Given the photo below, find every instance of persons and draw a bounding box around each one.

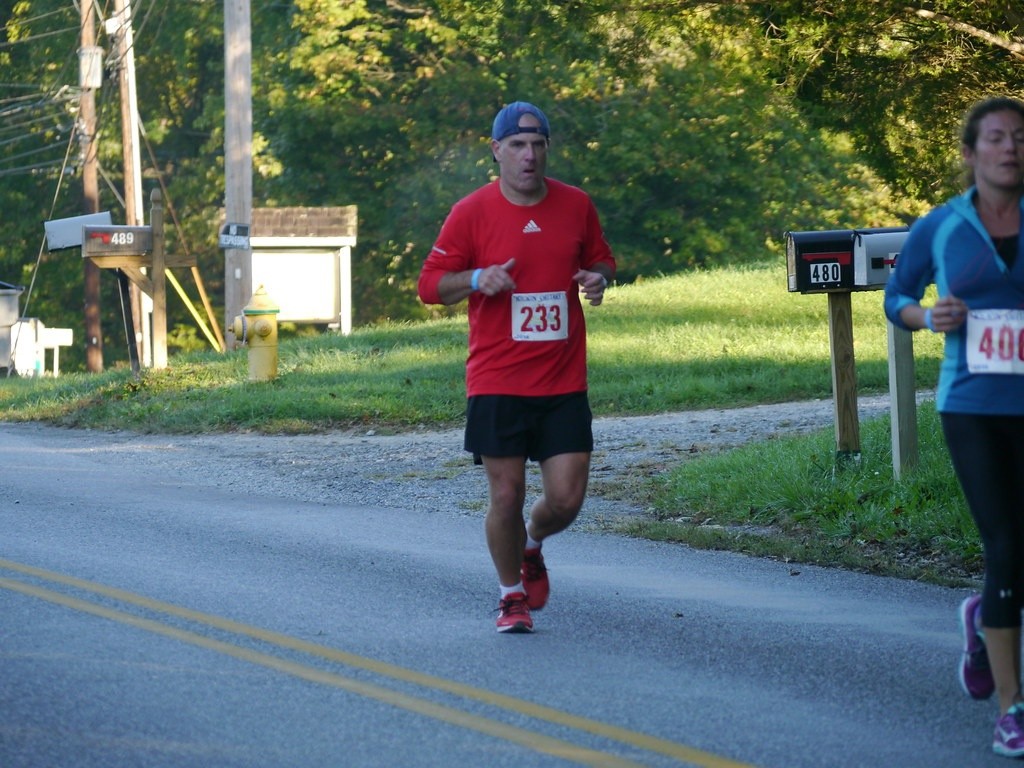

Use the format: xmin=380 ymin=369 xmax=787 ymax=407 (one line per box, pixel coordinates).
xmin=884 ymin=98 xmax=1024 ymax=756
xmin=417 ymin=102 xmax=618 ymax=634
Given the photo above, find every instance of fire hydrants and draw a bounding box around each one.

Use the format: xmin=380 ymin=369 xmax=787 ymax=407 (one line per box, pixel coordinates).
xmin=226 ymin=283 xmax=280 ymax=385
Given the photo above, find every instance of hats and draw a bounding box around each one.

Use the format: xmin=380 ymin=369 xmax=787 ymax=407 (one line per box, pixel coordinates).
xmin=491 ymin=101 xmax=549 ymax=163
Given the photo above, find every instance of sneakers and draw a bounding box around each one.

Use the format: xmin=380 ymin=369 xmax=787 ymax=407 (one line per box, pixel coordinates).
xmin=992 ymin=691 xmax=1024 ymax=757
xmin=959 ymin=595 xmax=996 ymax=700
xmin=520 ymin=547 xmax=550 ymax=611
xmin=489 ymin=592 xmax=533 ymax=633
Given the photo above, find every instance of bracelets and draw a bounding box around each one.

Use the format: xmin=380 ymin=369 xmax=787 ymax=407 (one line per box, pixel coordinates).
xmin=925 ymin=309 xmax=932 ymax=329
xmin=471 ymin=268 xmax=482 ymax=290
